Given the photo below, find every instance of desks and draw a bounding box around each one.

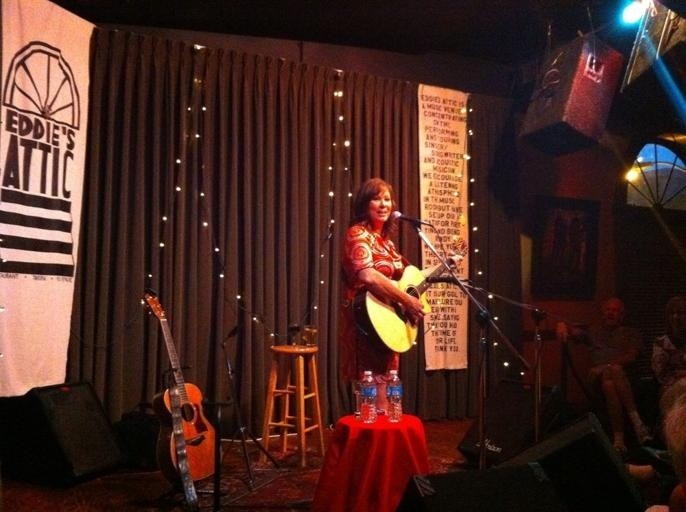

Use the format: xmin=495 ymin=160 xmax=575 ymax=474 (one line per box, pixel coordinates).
xmin=335 ymin=412 xmax=428 ymax=511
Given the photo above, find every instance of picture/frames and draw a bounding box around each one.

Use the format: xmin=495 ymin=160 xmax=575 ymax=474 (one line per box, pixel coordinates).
xmin=531 ymin=194 xmax=600 ymax=301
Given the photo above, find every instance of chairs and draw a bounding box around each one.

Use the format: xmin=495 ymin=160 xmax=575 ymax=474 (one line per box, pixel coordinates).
xmin=564 ymin=337 xmax=606 ymax=410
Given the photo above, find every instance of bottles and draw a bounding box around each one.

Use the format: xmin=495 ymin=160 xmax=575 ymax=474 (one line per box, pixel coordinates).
xmin=385 ymin=369 xmax=404 ymax=424
xmin=359 ymin=371 xmax=378 ymax=425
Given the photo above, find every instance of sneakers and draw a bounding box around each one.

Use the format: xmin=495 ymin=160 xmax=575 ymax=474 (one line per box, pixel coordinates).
xmin=611 ymin=427 xmax=654 ymax=466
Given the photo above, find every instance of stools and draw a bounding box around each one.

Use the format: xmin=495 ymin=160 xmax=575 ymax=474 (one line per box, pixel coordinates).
xmin=255 ymin=343 xmax=327 ymax=468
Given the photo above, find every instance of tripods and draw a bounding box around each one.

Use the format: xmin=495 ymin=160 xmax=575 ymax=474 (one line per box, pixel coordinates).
xmin=219 ymin=325 xmax=281 ymax=488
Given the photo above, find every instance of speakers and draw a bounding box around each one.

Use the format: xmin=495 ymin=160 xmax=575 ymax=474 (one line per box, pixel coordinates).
xmin=488 ymin=412 xmax=646 ymax=512
xmin=458 ymin=384 xmax=580 ymax=470
xmin=0 ymin=382 xmax=136 ymax=490
xmin=519 ymin=31 xmax=626 ymax=158
xmin=396 ymin=460 xmax=565 ymax=512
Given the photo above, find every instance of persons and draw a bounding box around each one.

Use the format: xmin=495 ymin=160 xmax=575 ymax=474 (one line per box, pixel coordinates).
xmin=568 ymin=295 xmax=686 ymax=512
xmin=338 ymin=178 xmax=426 ymax=412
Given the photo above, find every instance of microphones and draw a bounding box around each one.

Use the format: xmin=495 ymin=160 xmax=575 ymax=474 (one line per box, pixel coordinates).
xmin=426 ymin=276 xmax=456 ymax=282
xmin=391 ymin=210 xmax=420 ymax=223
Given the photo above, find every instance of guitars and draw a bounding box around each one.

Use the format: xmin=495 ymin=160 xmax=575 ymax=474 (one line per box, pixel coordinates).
xmin=140 ymin=287 xmax=223 ymax=487
xmin=351 ymin=237 xmax=468 ymax=354
